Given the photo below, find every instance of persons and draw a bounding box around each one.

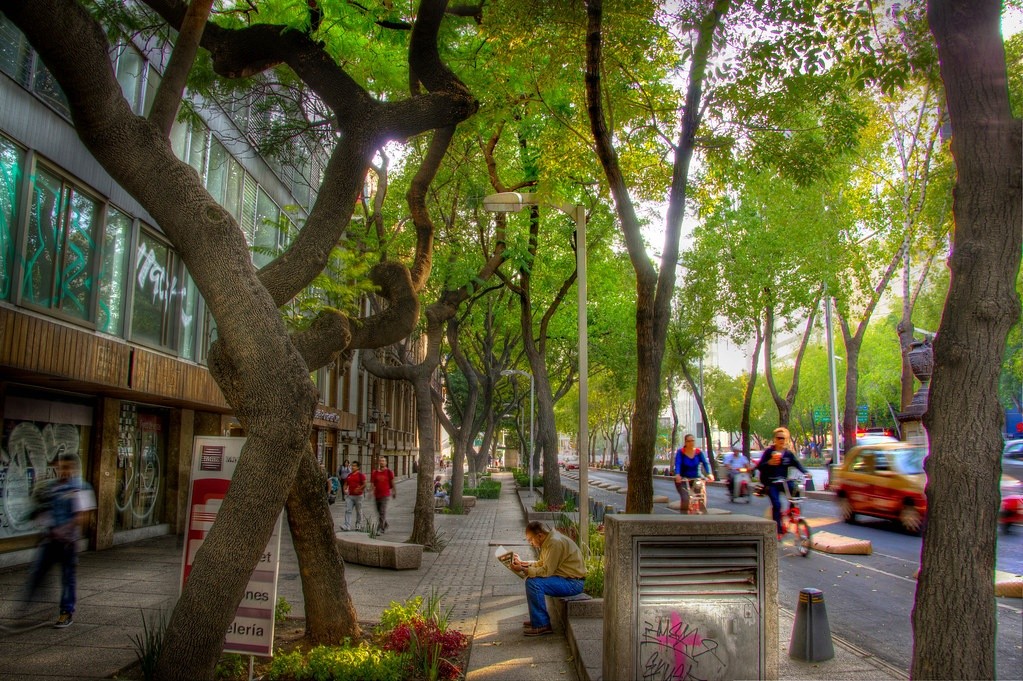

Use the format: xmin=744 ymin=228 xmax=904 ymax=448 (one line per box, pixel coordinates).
xmin=722 ymin=447 xmax=753 ymax=494
xmin=320 ymin=461 xmax=332 ymax=504
xmin=340 ymin=460 xmax=367 ymax=531
xmin=510 ymin=521 xmax=587 ymax=637
xmin=1 ymin=451 xmax=97 ymax=633
xmin=674 ymin=434 xmax=715 ymax=513
xmin=367 ymin=456 xmax=396 ymax=537
xmin=336 ymin=460 xmax=352 ymax=502
xmin=434 ymin=459 xmax=451 ymax=497
xmin=759 ymin=428 xmax=812 ymax=533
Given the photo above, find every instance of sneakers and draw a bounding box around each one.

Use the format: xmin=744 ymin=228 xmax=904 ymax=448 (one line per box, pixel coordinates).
xmin=56 ymin=608 xmax=74 ymax=627
xmin=523 ymin=618 xmax=552 ymax=636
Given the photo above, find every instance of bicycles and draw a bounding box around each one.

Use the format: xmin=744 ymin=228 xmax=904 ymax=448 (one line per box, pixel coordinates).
xmin=764 ymin=474 xmax=815 ymax=558
xmin=679 ymin=477 xmax=711 ymax=517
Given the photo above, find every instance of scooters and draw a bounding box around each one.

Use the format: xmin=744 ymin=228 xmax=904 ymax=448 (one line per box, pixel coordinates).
xmin=724 ymin=463 xmax=754 ymax=503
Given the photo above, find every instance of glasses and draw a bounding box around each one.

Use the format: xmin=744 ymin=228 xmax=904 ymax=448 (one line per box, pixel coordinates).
xmin=776 ymin=436 xmax=786 ymax=440
xmin=686 ymin=440 xmax=694 ymax=443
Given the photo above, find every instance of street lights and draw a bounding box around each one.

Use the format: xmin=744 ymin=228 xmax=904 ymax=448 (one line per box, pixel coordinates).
xmin=502 ymin=414 xmax=520 ymax=474
xmin=484 ymin=190 xmax=590 ymax=562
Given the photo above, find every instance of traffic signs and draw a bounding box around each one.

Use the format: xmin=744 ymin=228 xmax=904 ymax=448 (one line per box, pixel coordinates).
xmin=812 ymin=402 xmax=869 ymax=423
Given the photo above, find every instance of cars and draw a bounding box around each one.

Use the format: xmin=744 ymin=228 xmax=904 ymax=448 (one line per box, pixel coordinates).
xmin=855 ymin=435 xmax=901 ymax=469
xmin=562 ymin=455 xmax=579 ymax=471
xmin=748 ymin=450 xmax=767 ymax=471
xmin=1001 ymin=438 xmax=1023 ymax=486
xmin=558 ymin=456 xmax=566 ymax=467
xmin=829 ymin=441 xmax=1023 ymax=539
xmin=701 ymin=451 xmax=745 ymax=481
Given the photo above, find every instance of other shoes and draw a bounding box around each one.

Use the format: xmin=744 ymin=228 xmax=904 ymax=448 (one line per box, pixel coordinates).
xmin=355 ymin=523 xmax=360 ymax=531
xmin=340 ymin=523 xmax=349 ymax=530
xmin=376 ymin=522 xmax=389 ymax=536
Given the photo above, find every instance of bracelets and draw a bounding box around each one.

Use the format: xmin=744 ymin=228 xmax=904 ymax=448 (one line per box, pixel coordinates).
xmin=521 ymin=567 xmax=525 ymax=571
